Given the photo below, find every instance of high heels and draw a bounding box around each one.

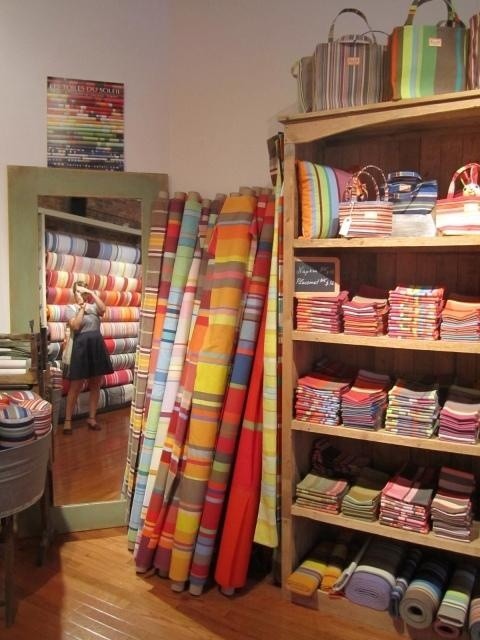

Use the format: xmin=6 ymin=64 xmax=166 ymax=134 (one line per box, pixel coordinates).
xmin=63 ymin=420 xmax=73 ymax=435
xmin=87 ymin=417 xmax=101 ymax=431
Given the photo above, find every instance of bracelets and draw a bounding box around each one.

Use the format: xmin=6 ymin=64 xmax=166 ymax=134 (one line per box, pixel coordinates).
xmin=77 ymin=313 xmax=84 ymax=316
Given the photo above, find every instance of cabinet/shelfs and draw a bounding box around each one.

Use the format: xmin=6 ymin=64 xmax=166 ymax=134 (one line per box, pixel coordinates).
xmin=279 ymin=88 xmax=480 ymax=637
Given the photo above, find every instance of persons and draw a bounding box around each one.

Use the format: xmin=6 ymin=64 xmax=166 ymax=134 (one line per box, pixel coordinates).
xmin=61 ymin=278 xmax=115 ymax=434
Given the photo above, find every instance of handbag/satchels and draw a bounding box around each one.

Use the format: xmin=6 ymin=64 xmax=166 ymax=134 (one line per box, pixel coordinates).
xmin=291 ymin=56 xmax=313 ymax=113
xmin=388 ymin=0 xmax=471 ymax=101
xmin=312 ymin=8 xmax=393 ymax=112
xmin=467 ymin=12 xmax=480 ymax=90
xmin=61 ymin=327 xmax=74 ymax=365
xmin=435 ymin=163 xmax=480 ymax=236
xmin=338 ymin=165 xmax=393 ymax=238
xmin=378 ymin=171 xmax=438 ymax=214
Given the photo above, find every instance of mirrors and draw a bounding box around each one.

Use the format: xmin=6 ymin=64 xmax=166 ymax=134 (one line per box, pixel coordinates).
xmin=7 ymin=164 xmax=169 ymax=536
